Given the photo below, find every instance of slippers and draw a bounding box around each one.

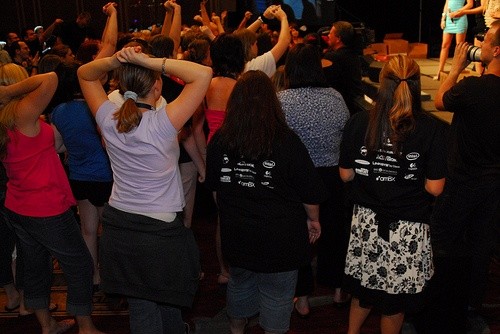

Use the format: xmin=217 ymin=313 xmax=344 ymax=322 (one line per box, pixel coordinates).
xmin=293 ymin=298 xmax=308 ymax=319
xmin=325 ymin=294 xmax=343 ymax=309
xmin=17 ymin=303 xmax=59 ymax=321
xmin=5 ymin=300 xmax=22 ymax=311
xmin=44 ymin=318 xmax=76 ymax=334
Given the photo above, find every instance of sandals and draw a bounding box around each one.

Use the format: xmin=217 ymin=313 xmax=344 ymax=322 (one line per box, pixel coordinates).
xmin=216 ymin=273 xmax=231 ymax=288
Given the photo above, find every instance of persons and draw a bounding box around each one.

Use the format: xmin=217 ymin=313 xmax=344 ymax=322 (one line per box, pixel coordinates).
xmin=0 ymin=0 xmax=500 ymax=334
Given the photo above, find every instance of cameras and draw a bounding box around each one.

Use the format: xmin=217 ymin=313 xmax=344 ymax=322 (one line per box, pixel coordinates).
xmin=466 ymin=32 xmax=486 ymax=62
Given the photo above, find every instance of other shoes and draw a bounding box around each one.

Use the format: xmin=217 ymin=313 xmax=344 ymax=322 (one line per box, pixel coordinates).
xmin=91 ymin=283 xmax=103 ymax=294
